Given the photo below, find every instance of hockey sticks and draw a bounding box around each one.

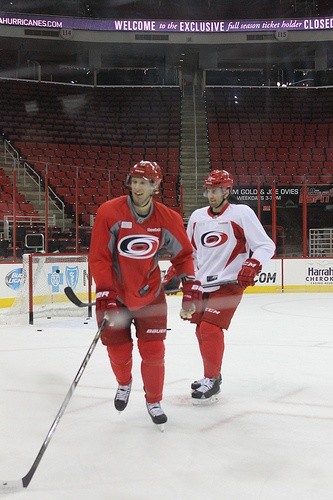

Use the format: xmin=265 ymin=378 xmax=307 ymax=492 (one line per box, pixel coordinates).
xmin=64 ymin=278 xmax=240 ymax=308
xmin=0 ymin=314 xmax=110 ymax=490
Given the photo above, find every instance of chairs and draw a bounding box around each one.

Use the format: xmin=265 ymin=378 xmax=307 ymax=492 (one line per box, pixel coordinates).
xmin=204 ymin=88 xmax=333 ymax=186
xmin=0 ymin=79 xmax=183 ymax=252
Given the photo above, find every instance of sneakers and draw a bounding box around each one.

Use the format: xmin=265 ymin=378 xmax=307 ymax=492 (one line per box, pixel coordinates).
xmin=114 ymin=376 xmax=134 ymax=413
xmin=192 ymin=378 xmax=222 ymax=404
xmin=146 ymin=400 xmax=168 ymax=431
xmin=190 ymin=373 xmax=223 ymax=390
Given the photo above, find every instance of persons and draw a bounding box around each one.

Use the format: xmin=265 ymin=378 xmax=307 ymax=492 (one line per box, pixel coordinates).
xmin=88 ymin=160 xmax=203 ymax=424
xmin=161 ymin=169 xmax=276 ymax=401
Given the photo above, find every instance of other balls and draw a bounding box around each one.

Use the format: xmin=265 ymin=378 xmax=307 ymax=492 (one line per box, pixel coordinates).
xmin=47 ymin=316 xmax=51 ymax=319
xmin=37 ymin=329 xmax=42 ymax=331
xmin=84 ymin=321 xmax=88 ymax=324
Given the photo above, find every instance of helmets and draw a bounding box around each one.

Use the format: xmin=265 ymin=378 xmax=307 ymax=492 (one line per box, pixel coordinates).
xmin=203 ymin=170 xmax=233 ymax=193
xmin=127 ymin=160 xmax=162 ymax=185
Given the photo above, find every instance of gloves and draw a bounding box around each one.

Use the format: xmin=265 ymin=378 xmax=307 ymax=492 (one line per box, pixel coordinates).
xmin=162 ymin=265 xmax=180 ymax=295
xmin=180 ymin=280 xmax=202 ymax=319
xmin=237 ymin=257 xmax=262 ymax=290
xmin=96 ymin=291 xmax=117 ymax=328
